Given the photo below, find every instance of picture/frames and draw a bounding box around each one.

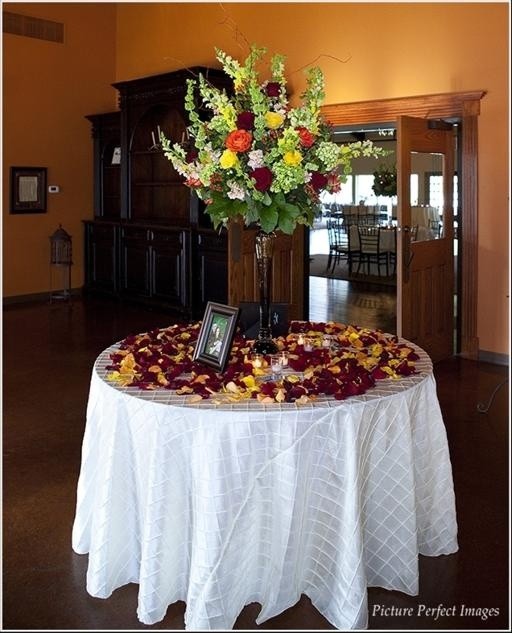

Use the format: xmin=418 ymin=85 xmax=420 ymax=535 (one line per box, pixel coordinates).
xmin=9 ymin=166 xmax=48 ymax=216
xmin=190 ymin=302 xmax=240 ymax=374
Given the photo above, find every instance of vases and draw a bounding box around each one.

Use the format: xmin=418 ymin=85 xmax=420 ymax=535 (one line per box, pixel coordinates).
xmin=253 ymin=230 xmax=278 ymax=352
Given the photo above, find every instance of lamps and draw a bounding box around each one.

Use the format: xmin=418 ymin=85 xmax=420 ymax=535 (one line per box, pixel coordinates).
xmin=47 ymin=226 xmax=81 ymax=304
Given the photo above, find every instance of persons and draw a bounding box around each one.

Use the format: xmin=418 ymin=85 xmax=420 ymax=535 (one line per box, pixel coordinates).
xmin=207 ymin=322 xmax=223 ymax=345
xmin=209 ymin=326 xmax=223 ymax=354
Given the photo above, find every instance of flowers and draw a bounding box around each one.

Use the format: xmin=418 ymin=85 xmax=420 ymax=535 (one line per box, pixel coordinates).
xmin=151 ymin=37 xmax=396 ymax=239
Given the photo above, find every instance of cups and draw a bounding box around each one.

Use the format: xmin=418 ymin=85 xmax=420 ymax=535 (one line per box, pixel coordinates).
xmin=251 ymin=350 xmax=290 ymax=374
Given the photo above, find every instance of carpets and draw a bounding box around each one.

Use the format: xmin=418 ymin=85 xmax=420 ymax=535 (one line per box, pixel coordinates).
xmin=308 ymin=253 xmax=395 ymax=286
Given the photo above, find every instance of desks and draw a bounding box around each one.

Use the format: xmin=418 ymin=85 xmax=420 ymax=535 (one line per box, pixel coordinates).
xmin=89 ymin=321 xmax=442 ymax=628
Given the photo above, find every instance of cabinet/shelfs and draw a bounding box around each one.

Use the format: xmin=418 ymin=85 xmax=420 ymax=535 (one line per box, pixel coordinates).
xmin=79 ymin=66 xmax=234 ymax=316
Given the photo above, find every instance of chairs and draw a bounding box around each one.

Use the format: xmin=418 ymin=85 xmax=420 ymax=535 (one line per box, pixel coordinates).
xmin=315 ymin=199 xmax=442 ymax=278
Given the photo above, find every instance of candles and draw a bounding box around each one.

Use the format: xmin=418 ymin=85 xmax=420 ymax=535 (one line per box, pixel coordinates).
xmin=294 ymin=330 xmax=332 ymax=351
xmin=252 ymin=350 xmax=292 ymax=374
